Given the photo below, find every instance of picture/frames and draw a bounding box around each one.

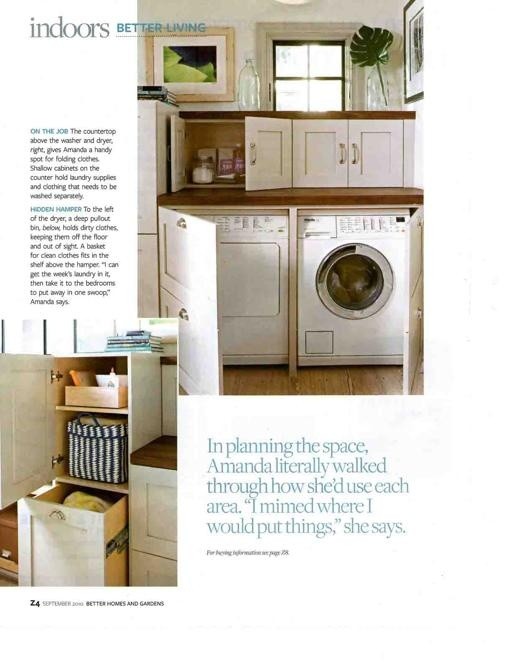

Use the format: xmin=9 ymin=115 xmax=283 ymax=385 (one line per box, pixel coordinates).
xmin=400 ymin=0 xmax=425 ymax=106
xmin=143 ymin=21 xmax=238 ymax=104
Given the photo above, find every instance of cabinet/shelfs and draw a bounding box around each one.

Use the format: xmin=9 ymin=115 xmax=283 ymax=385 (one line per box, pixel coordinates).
xmin=126 ymin=467 xmax=177 ymax=587
xmin=0 ymin=351 xmax=164 ymax=511
xmin=164 ymin=112 xmax=292 ymax=193
xmin=157 ymin=203 xmax=425 ymax=396
xmin=15 ymin=482 xmax=129 ymax=587
xmin=290 ymin=118 xmax=405 ymax=189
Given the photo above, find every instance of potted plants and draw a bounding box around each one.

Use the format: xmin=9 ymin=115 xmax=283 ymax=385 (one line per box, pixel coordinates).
xmin=348 ymin=23 xmax=395 ymax=110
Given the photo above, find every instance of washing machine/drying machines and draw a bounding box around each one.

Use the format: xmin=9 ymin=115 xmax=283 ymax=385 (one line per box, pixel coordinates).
xmin=159 ymin=208 xmax=291 ymax=394
xmin=298 ymin=212 xmax=411 ymax=369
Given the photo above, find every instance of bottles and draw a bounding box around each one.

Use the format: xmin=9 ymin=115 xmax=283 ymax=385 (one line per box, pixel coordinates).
xmin=192 ymin=154 xmax=215 ymax=184
xmin=233 ymin=144 xmax=246 ymax=184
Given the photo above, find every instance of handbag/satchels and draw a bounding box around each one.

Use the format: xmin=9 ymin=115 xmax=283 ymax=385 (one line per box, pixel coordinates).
xmin=66 ymin=411 xmax=128 ymax=485
